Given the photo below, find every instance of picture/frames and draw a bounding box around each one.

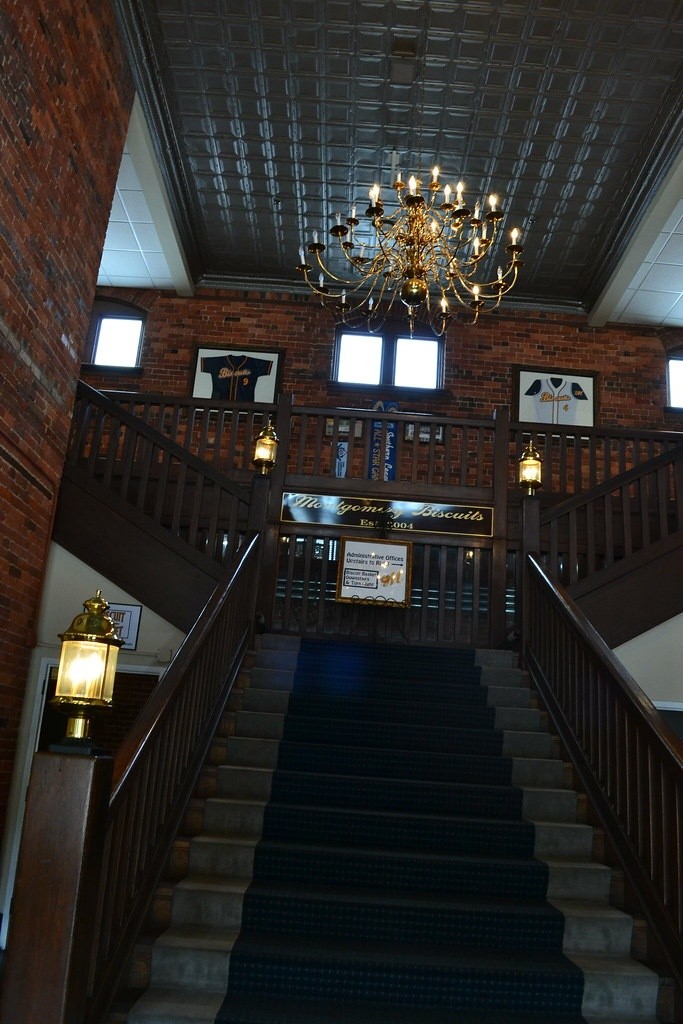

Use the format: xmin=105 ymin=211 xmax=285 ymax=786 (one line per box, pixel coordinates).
xmin=83 ymin=602 xmax=142 ymax=651
xmin=181 ymin=342 xmax=288 ymax=426
xmin=511 ymin=362 xmax=602 ymax=447
xmin=334 ymin=536 xmax=414 ymax=609
xmin=403 ymin=411 xmax=446 ymax=445
xmin=322 ymin=407 xmax=366 ymax=441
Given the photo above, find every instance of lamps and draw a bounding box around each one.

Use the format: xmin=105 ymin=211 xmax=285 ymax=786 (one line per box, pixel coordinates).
xmin=252 ymin=419 xmax=280 ymax=476
xmin=296 ymin=2 xmax=527 ymax=340
xmin=49 ymin=588 xmax=127 ymax=756
xmin=517 ymin=440 xmax=547 ymax=497
xmin=464 ymin=549 xmax=474 ymax=569
